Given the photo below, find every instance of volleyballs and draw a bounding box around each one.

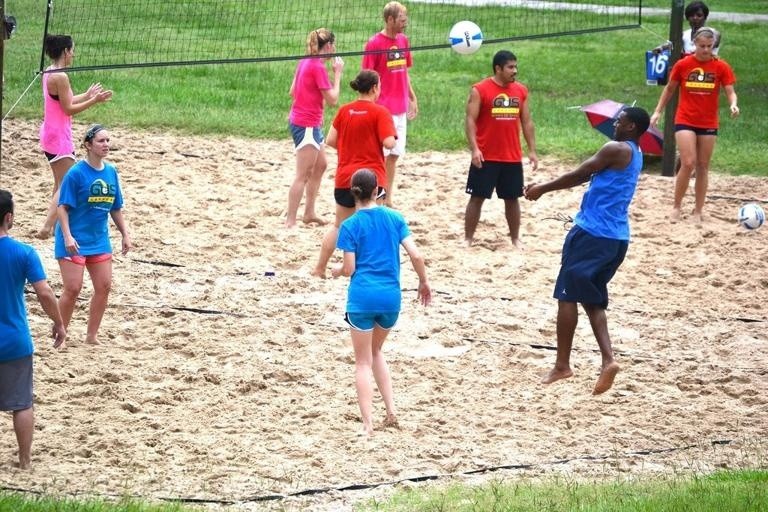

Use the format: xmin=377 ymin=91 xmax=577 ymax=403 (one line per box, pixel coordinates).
xmin=449 ymin=20 xmax=484 ymax=56
xmin=738 ymin=203 xmax=765 ymax=230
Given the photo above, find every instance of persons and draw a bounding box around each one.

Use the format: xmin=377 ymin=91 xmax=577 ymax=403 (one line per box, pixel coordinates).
xmin=331 ymin=167 xmax=432 ymax=438
xmin=35 ymin=33 xmax=113 ymax=237
xmin=463 ymin=50 xmax=538 ymax=253
xmin=521 ymin=104 xmax=649 ymax=397
xmin=363 ymin=1 xmax=418 ymax=208
xmin=652 ymin=2 xmax=721 ymax=175
xmin=284 ymin=27 xmax=345 ymax=231
xmin=0 ymin=189 xmax=67 ymax=470
xmin=49 ymin=122 xmax=131 ymax=346
xmin=308 ymin=69 xmax=398 ymax=289
xmin=649 ymin=26 xmax=740 ymax=225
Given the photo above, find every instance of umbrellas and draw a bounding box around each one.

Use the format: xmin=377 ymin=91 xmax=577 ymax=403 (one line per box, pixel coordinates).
xmin=578 ymin=98 xmax=665 ymax=157
